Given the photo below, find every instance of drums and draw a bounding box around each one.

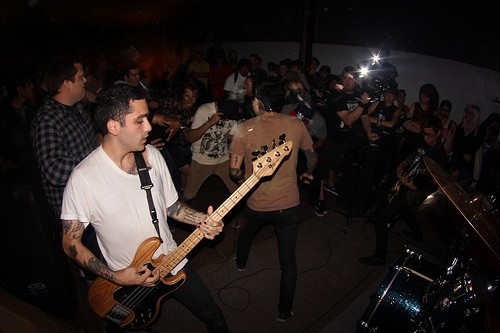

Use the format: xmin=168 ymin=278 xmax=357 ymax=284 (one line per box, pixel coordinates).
xmin=425 ymin=260 xmax=496 ymax=333
xmin=358 ymin=248 xmax=450 ymax=333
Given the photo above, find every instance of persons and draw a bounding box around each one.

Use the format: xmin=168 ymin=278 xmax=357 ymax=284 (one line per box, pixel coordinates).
xmin=31 ymin=61 xmax=107 ymax=333
xmin=311 ymin=47 xmax=500 ymax=217
xmin=60 ymin=83 xmax=224 ymax=333
xmin=227 ymin=77 xmax=317 ymax=323
xmin=112 ymin=55 xmax=331 ymax=211
xmin=0 ymin=53 xmax=105 ymax=271
xmin=182 ymin=79 xmax=238 ymax=202
xmin=359 ymin=115 xmax=446 ymax=265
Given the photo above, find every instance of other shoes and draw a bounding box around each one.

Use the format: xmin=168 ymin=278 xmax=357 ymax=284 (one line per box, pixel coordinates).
xmin=276 ymin=311 xmax=294 ymax=322
xmin=234 ymin=257 xmax=247 ymax=272
xmin=360 ymin=255 xmax=384 ymax=264
xmin=312 ymin=205 xmax=327 ymax=216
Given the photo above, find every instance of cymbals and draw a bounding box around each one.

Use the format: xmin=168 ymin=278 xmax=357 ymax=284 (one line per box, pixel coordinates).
xmin=423 ymin=155 xmax=500 ymax=260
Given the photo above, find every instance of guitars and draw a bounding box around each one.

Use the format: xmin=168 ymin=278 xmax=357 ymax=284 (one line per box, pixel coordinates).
xmin=89 ymin=133 xmax=294 ymax=329
xmin=364 ymin=149 xmax=427 ymax=219
